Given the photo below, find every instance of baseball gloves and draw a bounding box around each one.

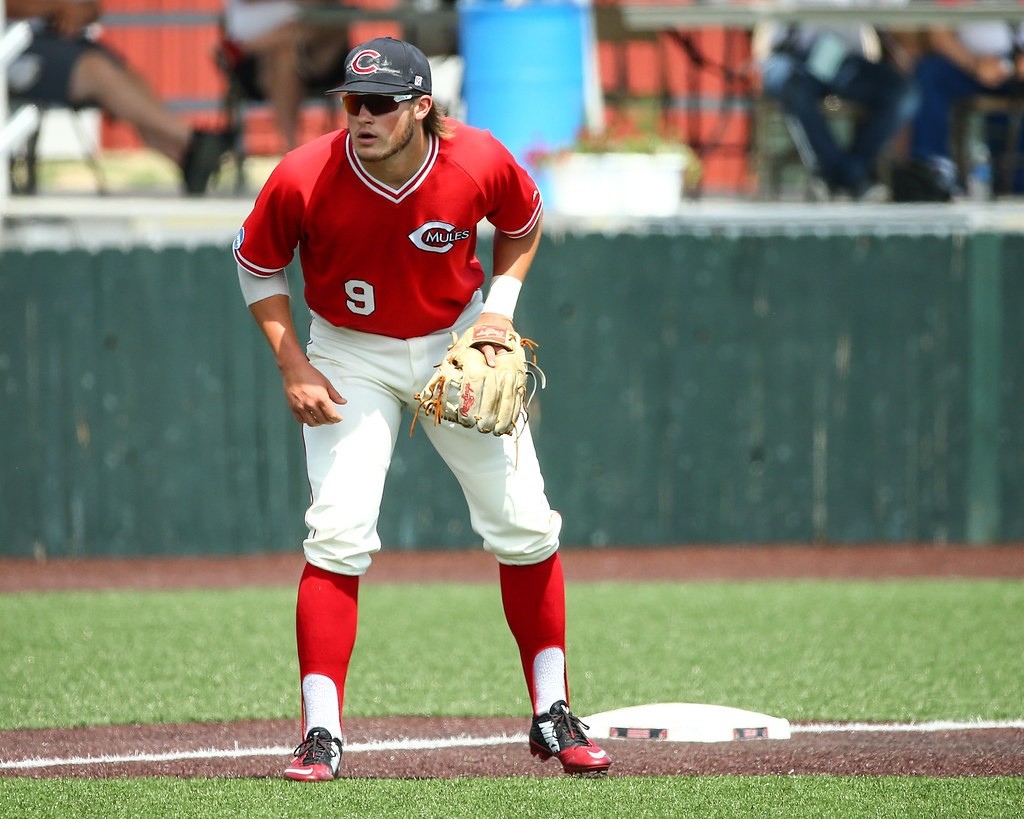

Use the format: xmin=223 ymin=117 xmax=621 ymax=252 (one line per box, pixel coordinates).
xmin=420 ymin=324 xmax=529 ymax=437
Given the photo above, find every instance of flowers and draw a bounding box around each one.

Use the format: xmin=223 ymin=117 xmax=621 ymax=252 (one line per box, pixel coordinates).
xmin=529 ymin=102 xmax=696 ymax=181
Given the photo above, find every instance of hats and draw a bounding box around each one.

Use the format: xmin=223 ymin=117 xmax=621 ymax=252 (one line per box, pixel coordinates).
xmin=324 ymin=37 xmax=432 ymax=97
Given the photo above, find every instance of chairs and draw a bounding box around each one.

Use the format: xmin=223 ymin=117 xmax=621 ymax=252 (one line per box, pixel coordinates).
xmin=9 ymin=90 xmax=105 ymax=199
xmin=221 ymin=85 xmax=343 ymax=201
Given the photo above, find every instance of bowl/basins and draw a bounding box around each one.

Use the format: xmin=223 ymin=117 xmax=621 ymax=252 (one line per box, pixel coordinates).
xmin=544 ymin=151 xmax=683 ymax=221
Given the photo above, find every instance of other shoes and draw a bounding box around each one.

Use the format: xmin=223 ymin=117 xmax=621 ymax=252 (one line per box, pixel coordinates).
xmin=180 ymin=121 xmax=239 ymax=194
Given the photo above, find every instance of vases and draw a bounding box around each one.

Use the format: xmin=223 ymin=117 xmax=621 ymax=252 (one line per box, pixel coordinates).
xmin=556 ymin=152 xmax=693 ymax=219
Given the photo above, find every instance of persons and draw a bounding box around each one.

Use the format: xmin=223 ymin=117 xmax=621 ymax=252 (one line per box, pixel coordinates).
xmin=750 ymin=0 xmax=1023 ymax=204
xmin=232 ymin=37 xmax=611 ymax=782
xmin=5 ymin=2 xmax=459 ymax=195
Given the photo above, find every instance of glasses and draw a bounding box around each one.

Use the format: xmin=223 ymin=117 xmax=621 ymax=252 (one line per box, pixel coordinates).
xmin=341 ymin=93 xmax=426 ymax=116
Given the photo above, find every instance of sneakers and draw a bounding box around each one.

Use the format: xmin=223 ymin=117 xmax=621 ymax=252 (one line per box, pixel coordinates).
xmin=283 ymin=727 xmax=343 ymax=782
xmin=529 ymin=699 xmax=612 ymax=776
